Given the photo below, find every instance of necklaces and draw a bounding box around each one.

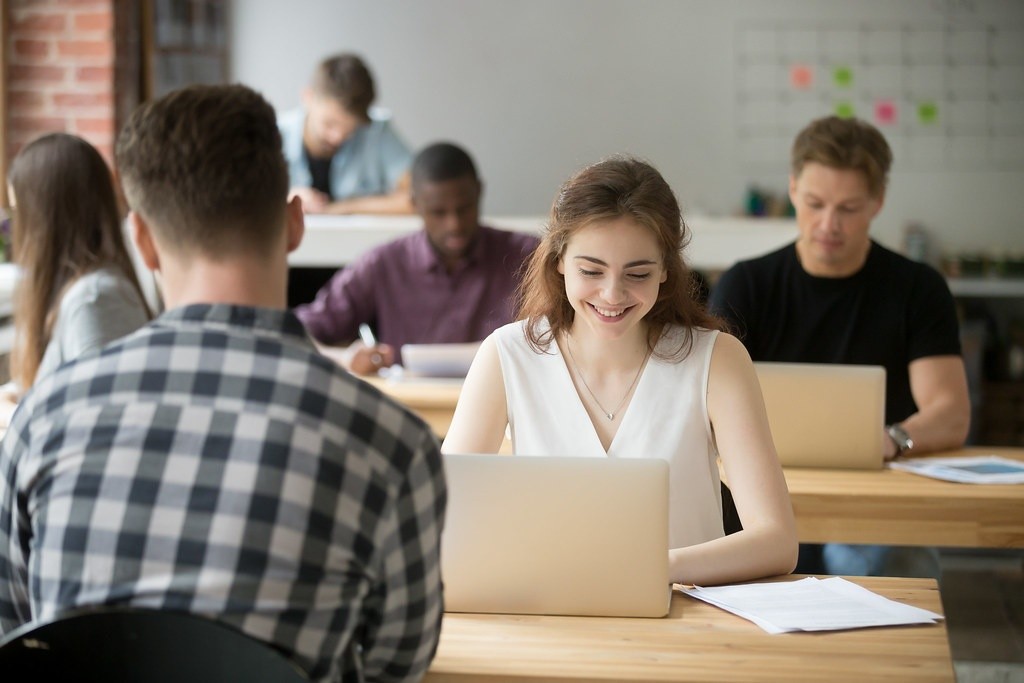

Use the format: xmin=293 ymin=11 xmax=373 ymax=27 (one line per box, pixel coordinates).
xmin=567 ymin=329 xmax=649 ymax=420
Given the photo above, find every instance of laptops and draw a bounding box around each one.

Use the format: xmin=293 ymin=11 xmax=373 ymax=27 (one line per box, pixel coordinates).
xmin=439 ymin=454 xmax=673 ymax=618
xmin=752 ymin=361 xmax=888 ymax=469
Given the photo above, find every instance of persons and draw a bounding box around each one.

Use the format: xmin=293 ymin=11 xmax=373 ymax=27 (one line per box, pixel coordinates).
xmin=276 ymin=55 xmax=416 ymax=215
xmin=712 ymin=115 xmax=970 ymax=575
xmin=0 ymin=83 xmax=447 ymax=683
xmin=8 ymin=133 xmax=151 ymax=403
xmin=439 ymin=157 xmax=799 ymax=583
xmin=297 ymin=141 xmax=541 ymax=378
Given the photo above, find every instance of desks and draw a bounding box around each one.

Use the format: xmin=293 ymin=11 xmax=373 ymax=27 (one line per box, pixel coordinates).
xmin=289 ymin=216 xmax=1024 ymax=683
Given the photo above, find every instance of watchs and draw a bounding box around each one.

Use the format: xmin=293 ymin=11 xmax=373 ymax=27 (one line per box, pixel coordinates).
xmin=884 ymin=425 xmax=910 ymax=462
